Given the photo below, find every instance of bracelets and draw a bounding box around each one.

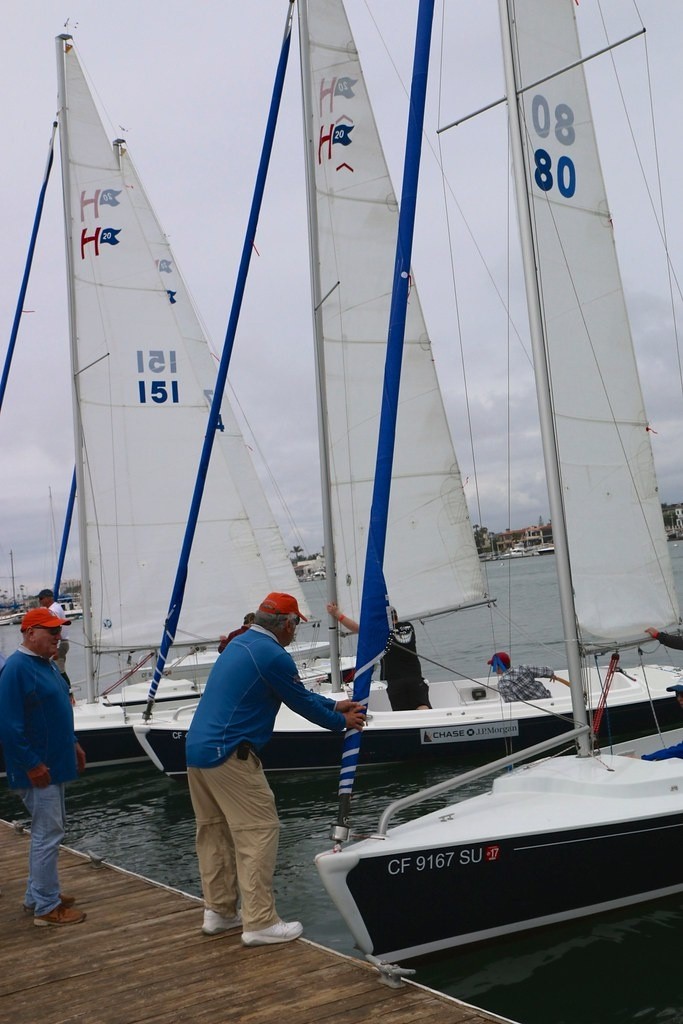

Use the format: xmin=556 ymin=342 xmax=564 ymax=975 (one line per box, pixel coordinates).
xmin=338 ymin=614 xmax=345 ymax=621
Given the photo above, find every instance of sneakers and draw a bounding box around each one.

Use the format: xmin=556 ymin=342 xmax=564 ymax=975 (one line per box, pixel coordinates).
xmin=241 ymin=918 xmax=303 ymax=946
xmin=23 ymin=894 xmax=75 ymax=913
xmin=202 ymin=909 xmax=242 ymax=934
xmin=34 ymin=907 xmax=86 ymax=926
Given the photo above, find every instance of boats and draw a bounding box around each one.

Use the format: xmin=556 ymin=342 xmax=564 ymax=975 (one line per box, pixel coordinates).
xmin=537 ymin=546 xmax=554 ymax=556
xmin=500 ymin=553 xmax=510 ymax=559
xmin=509 ymin=546 xmax=532 ymax=557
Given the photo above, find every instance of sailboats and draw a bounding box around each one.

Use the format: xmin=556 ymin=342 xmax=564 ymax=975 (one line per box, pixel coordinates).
xmin=1 ymin=0 xmax=683 ymax=972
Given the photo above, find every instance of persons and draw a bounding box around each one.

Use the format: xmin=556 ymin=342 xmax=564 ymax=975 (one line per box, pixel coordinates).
xmin=644 ymin=626 xmax=683 ymax=650
xmin=187 ymin=592 xmax=368 ymax=945
xmin=487 ymin=652 xmax=555 ymax=702
xmin=628 ymin=676 xmax=683 ymax=762
xmin=327 ymin=602 xmax=432 ymax=711
xmin=35 ymin=590 xmax=75 ymax=705
xmin=0 ymin=608 xmax=86 ymax=928
xmin=218 ymin=613 xmax=255 ymax=653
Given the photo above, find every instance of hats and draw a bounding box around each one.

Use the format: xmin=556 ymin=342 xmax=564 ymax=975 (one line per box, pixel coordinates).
xmin=487 ymin=652 xmax=510 ymax=665
xmin=259 ymin=593 xmax=308 ymax=623
xmin=20 ymin=608 xmax=72 ymax=631
xmin=33 ymin=589 xmax=54 ymax=598
xmin=666 ymin=676 xmax=683 ymax=692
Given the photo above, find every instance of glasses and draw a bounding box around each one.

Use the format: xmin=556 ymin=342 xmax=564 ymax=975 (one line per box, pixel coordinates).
xmin=32 ymin=626 xmax=62 ymax=635
xmin=39 ymin=597 xmax=45 ymax=600
